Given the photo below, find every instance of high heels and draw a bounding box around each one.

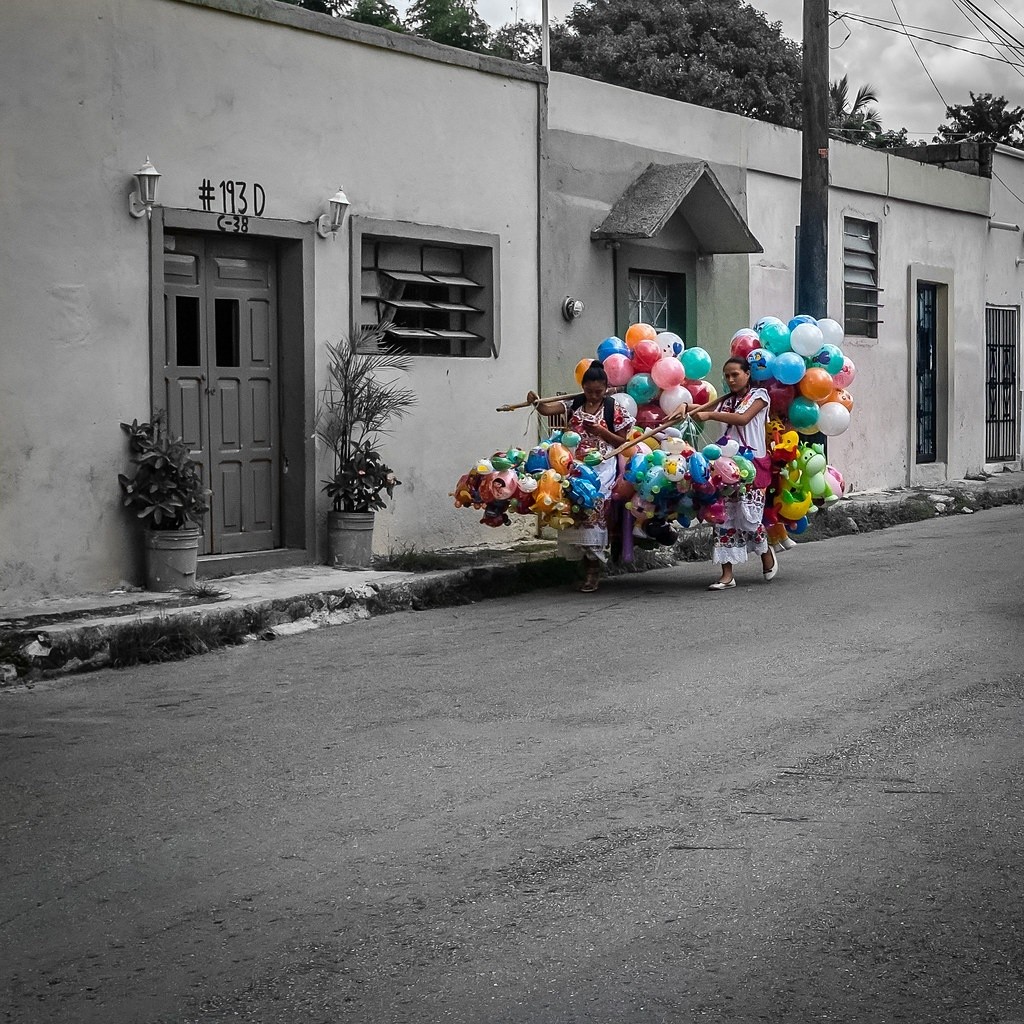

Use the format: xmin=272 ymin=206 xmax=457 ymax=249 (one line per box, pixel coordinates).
xmin=574 ymin=567 xmax=601 ymax=593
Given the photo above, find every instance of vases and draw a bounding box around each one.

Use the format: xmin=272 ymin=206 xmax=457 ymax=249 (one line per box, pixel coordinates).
xmin=146 ymin=529 xmax=200 ymax=592
xmin=327 ymin=510 xmax=375 ymax=569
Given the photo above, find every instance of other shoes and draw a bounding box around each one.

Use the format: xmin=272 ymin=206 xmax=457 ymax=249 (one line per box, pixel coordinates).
xmin=763 ymin=546 xmax=779 ymax=581
xmin=708 ymin=577 xmax=737 ymax=590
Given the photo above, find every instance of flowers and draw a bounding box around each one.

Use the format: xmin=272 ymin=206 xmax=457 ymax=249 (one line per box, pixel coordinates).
xmin=117 ymin=407 xmax=214 ymax=530
xmin=308 ymin=322 xmax=419 ymax=512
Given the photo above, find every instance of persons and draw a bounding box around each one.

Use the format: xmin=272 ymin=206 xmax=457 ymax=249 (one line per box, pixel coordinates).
xmin=663 ymin=356 xmax=778 ymax=590
xmin=526 ymin=360 xmax=636 ymax=592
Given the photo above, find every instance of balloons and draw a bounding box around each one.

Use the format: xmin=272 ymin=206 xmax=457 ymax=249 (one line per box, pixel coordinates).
xmin=729 ymin=313 xmax=857 ymax=436
xmin=575 ymin=322 xmax=717 ymax=435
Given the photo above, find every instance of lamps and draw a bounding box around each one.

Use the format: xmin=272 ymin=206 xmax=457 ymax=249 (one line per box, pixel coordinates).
xmin=316 ymin=183 xmax=350 ymax=238
xmin=129 ymin=154 xmax=162 ymax=219
xmin=563 ymin=295 xmax=585 ymax=322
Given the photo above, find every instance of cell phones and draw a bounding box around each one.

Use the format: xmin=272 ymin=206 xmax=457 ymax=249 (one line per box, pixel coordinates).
xmin=583 ymin=420 xmax=594 ymax=430
